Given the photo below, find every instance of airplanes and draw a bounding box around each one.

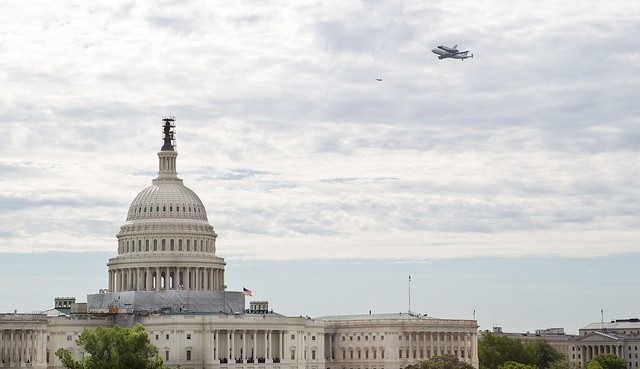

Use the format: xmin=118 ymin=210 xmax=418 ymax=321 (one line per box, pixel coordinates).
xmin=432 ymin=44 xmax=473 ymax=61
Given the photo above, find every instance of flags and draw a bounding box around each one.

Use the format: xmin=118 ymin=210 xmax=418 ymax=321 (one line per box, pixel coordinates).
xmin=243 ymin=287 xmax=252 ymax=296
